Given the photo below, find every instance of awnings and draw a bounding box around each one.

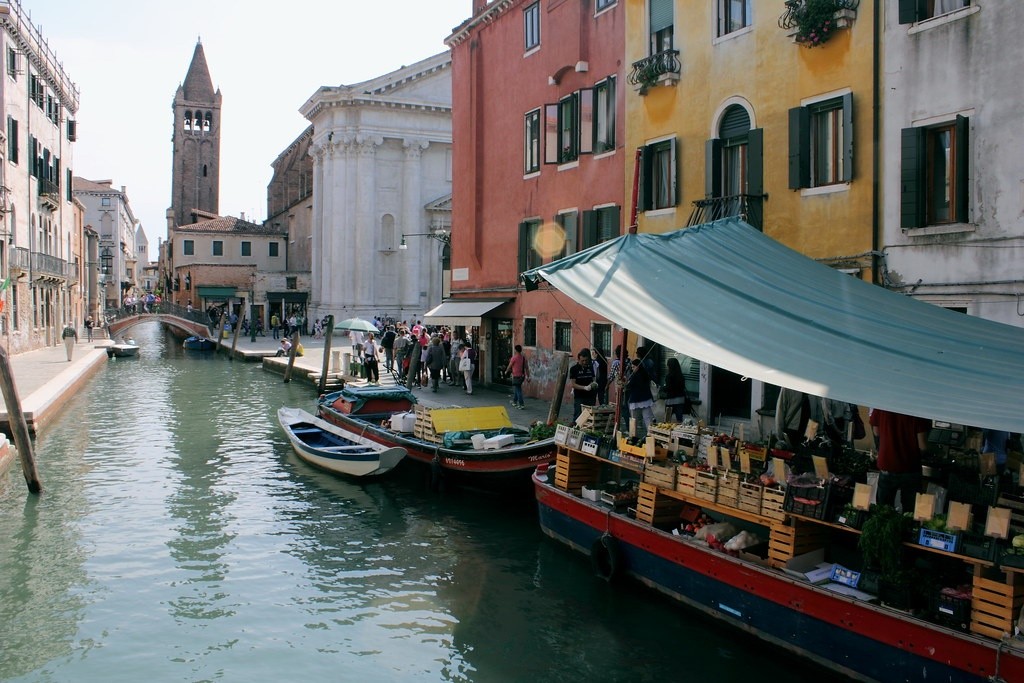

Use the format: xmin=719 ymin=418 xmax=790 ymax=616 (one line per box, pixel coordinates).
xmin=422 ymin=302 xmax=505 ymax=327
xmin=196 ymin=286 xmax=235 ymax=299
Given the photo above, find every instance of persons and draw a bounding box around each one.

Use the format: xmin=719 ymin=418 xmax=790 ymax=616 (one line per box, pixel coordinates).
xmin=84 ymin=292 xmax=329 ymax=358
xmin=504 ymin=345 xmax=531 ymax=410
xmin=568 ymin=345 xmax=686 ymax=442
xmin=348 ymin=315 xmax=477 ymax=396
xmin=775 ymin=387 xmax=1024 ymax=520
xmin=62 ymin=322 xmax=78 ymax=361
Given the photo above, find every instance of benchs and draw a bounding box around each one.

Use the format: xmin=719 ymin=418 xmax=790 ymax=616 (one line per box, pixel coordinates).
xmin=293 ymin=429 xmax=323 ymax=434
xmin=317 ymin=445 xmax=371 ymax=451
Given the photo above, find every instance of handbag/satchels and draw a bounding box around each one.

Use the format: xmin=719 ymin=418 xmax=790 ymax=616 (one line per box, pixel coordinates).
xmin=850 ymin=413 xmax=866 ymax=439
xmin=365 ymin=353 xmax=371 ymax=358
xmin=682 ymin=401 xmax=691 ymax=414
xmin=512 ymin=376 xmax=524 ymax=386
xmin=426 ymin=346 xmax=432 ymax=366
xmin=458 ymin=351 xmax=470 ymax=370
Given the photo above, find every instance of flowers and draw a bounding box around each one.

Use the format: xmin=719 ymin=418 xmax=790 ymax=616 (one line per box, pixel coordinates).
xmin=792 ymin=0 xmax=849 ymax=50
xmin=636 ymin=62 xmax=665 ymax=94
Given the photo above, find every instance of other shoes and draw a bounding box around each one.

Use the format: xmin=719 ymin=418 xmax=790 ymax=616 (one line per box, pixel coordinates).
xmin=515 ymin=405 xmax=524 ymax=409
xmin=440 ymin=380 xmax=446 ymax=383
xmin=462 ymin=386 xmax=474 ymax=395
xmin=417 ymin=384 xmax=421 ymax=389
xmin=431 ymin=385 xmax=439 ymax=392
xmin=510 ymin=400 xmax=517 ymax=407
xmin=446 ymin=381 xmax=460 ymax=386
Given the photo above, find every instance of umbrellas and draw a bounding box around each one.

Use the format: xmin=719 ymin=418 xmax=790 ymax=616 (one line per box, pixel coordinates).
xmin=334 ymin=316 xmax=381 ymax=363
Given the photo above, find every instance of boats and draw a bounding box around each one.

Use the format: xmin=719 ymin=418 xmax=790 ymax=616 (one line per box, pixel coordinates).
xmin=277 ymin=403 xmax=408 ymax=478
xmin=106 ymin=344 xmax=139 ymax=358
xmin=318 ymin=386 xmax=557 ymax=477
xmin=531 ymin=406 xmax=1024 ymax=682
xmin=185 ymin=336 xmax=214 ymax=350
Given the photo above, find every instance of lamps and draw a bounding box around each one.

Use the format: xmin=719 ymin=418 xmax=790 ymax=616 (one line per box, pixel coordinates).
xmin=398 ymin=233 xmax=451 ymax=250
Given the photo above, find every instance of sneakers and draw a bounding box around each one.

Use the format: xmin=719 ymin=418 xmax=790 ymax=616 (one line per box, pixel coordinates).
xmin=368 ymin=381 xmax=383 ymax=386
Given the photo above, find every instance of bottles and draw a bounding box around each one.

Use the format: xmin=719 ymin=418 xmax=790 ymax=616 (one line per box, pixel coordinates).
xmin=675 ymin=449 xmax=717 ymax=480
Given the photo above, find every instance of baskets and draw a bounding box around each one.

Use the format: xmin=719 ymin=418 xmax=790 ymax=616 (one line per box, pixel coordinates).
xmin=553 ymin=424 xmax=615 ymax=458
xmin=833 ymin=504 xmax=1024 ymax=575
xmin=946 ymin=472 xmax=1003 ymax=506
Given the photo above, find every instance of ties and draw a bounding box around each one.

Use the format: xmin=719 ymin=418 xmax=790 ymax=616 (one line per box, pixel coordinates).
xmin=797 ymin=393 xmax=810 ymax=437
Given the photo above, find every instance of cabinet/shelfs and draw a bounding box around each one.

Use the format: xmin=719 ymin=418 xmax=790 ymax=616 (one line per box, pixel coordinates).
xmin=580 ymin=401 xmax=617 ymax=433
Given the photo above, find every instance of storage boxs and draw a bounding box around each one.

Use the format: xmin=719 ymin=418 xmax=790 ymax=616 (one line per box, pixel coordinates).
xmin=391 ymin=413 xmax=417 ymax=433
xmin=552 ymin=424 xmax=854 ymax=522
xmin=919 ymin=528 xmax=1024 ymax=568
xmin=484 ymin=434 xmax=515 ymax=450
